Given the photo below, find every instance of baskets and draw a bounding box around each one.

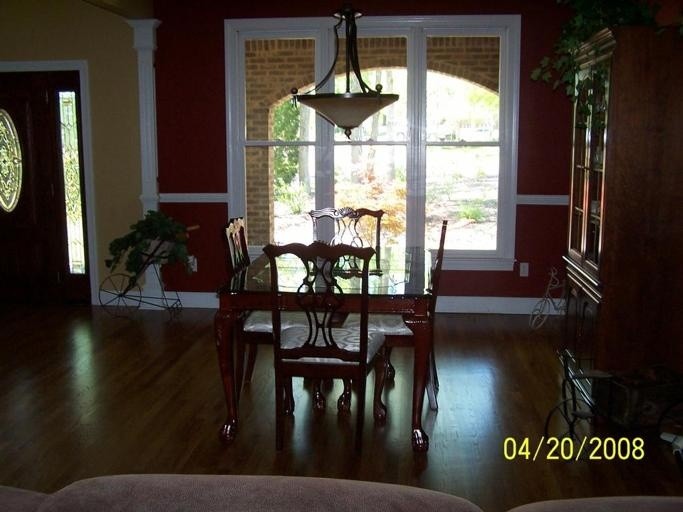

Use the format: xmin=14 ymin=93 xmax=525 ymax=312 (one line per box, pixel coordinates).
xmin=141 ymin=239 xmax=176 ymax=264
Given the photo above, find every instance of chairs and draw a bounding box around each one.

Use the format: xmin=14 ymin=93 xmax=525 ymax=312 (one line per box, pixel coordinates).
xmin=309 ymin=207 xmax=384 ymax=270
xmin=263 ymin=242 xmax=388 ymax=451
xmin=224 ymin=216 xmax=322 ymax=414
xmin=342 ymin=220 xmax=448 ymax=411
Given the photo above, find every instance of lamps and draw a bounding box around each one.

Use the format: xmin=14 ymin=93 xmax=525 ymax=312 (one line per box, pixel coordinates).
xmin=290 ymin=8 xmax=403 ymax=137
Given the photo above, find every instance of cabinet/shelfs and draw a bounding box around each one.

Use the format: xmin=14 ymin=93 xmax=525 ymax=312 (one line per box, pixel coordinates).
xmin=556 ymin=23 xmax=673 ymax=416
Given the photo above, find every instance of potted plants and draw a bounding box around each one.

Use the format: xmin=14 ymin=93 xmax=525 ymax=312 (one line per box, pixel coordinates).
xmin=105 ymin=210 xmax=196 ymax=283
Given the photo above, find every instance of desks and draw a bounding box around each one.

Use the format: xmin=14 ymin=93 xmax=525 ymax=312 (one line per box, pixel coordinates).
xmin=212 ymin=242 xmax=439 ymax=451
